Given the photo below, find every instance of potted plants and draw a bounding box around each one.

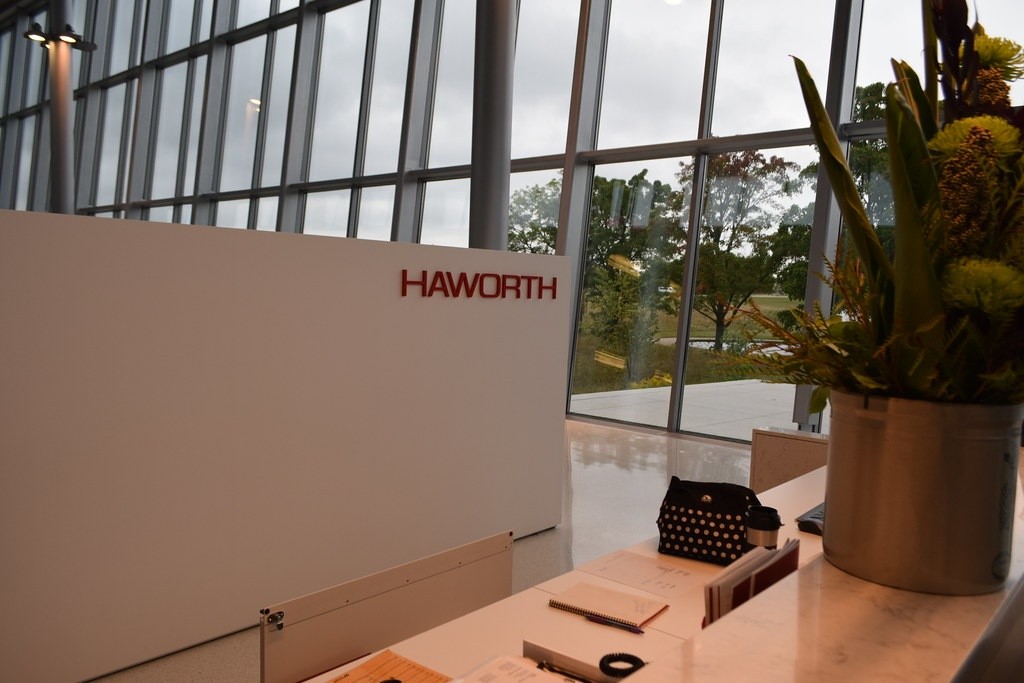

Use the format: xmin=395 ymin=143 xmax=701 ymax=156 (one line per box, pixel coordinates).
xmin=690 ymin=0 xmax=1024 ymax=597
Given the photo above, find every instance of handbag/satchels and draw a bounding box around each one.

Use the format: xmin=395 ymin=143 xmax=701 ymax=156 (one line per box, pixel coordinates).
xmin=656 ymin=476 xmax=763 ymax=567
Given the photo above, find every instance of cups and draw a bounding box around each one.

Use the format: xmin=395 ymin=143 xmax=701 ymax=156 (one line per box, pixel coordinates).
xmin=744 ymin=505 xmax=780 ymax=553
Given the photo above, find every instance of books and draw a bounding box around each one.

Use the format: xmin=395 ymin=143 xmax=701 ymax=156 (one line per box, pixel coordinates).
xmin=550 ymin=580 xmax=670 ymax=628
